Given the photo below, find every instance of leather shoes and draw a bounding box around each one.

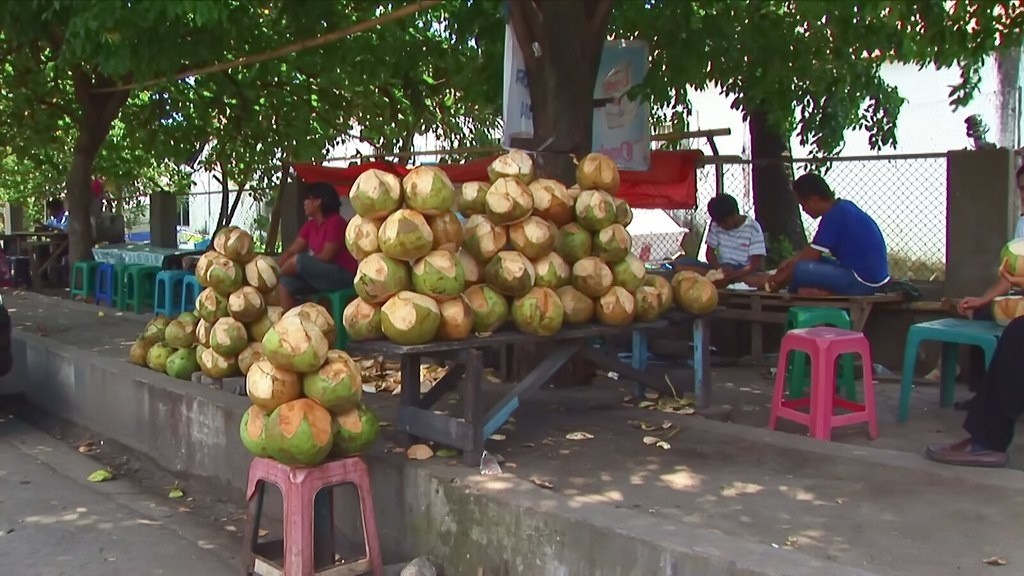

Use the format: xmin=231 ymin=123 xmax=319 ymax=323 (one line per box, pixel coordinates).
xmin=925 ymin=438 xmax=1010 ymax=467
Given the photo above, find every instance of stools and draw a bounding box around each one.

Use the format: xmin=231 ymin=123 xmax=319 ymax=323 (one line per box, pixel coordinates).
xmin=897 ymin=317 xmax=1006 ymax=423
xmin=240 ymin=456 xmax=383 ymax=576
xmin=70 ymin=259 xmax=204 ymax=320
xmin=770 ymin=327 xmax=879 ymax=440
xmin=785 ymin=306 xmax=858 ymax=405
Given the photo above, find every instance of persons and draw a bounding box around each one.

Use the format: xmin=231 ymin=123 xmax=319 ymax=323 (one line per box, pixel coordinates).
xmin=924 ymin=165 xmax=1024 ymax=384
xmin=767 ymin=171 xmax=891 ymax=296
xmin=33 ymin=198 xmax=70 ymax=280
xmin=277 ymin=181 xmax=357 ymax=313
xmin=673 ymin=193 xmax=767 ymax=287
xmin=928 ymin=258 xmax=1024 ymax=468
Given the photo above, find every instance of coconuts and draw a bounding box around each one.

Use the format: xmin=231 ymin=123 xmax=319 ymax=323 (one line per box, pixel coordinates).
xmin=342 ymin=149 xmax=719 ymax=346
xmin=1000 ymin=237 xmax=1024 ymax=277
xmin=129 ymin=226 xmax=336 ymax=381
xmin=991 ymin=295 xmax=1024 ymax=326
xmin=239 ymin=315 xmax=379 ymax=467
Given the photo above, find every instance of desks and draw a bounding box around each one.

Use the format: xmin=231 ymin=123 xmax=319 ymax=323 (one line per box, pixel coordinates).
xmin=92 ymin=244 xmax=207 ymax=272
xmin=713 ymin=284 xmax=908 ymax=375
xmin=12 ymin=231 xmax=68 ymax=278
xmin=347 ymin=306 xmax=728 ymax=472
